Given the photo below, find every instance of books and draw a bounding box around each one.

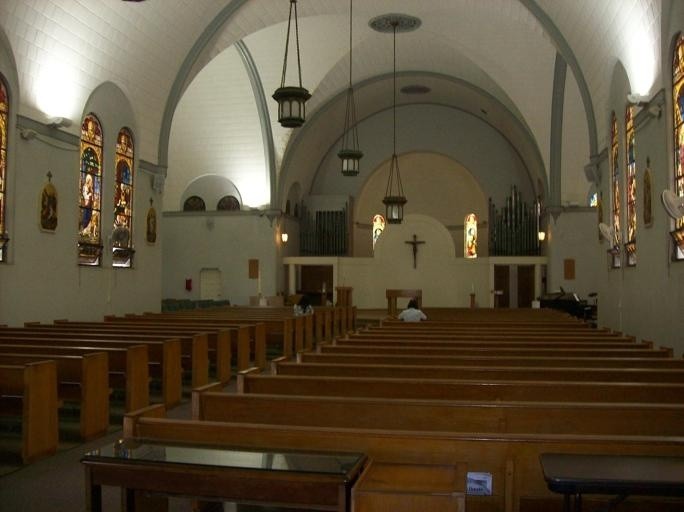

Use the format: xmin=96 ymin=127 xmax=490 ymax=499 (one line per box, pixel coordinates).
xmin=467 ymin=470 xmax=494 ymax=496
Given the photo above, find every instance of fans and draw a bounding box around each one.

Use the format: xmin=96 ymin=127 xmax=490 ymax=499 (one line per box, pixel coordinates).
xmin=598 ymin=222 xmax=620 ymax=244
xmin=660 ymin=189 xmax=683 ymax=219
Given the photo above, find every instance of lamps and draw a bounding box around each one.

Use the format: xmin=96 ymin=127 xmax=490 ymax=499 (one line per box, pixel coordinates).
xmin=269 ymin=0 xmax=406 ymax=230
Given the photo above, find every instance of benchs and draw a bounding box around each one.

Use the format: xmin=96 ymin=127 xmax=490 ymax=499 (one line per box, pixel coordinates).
xmin=1 ymin=305 xmax=684 ymax=507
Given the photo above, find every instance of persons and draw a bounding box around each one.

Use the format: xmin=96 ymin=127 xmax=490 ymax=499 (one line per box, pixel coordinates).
xmin=396 ymin=298 xmax=429 ymax=323
xmin=78 ymin=172 xmax=131 ymax=241
xmin=292 ymin=296 xmax=313 ymax=317
